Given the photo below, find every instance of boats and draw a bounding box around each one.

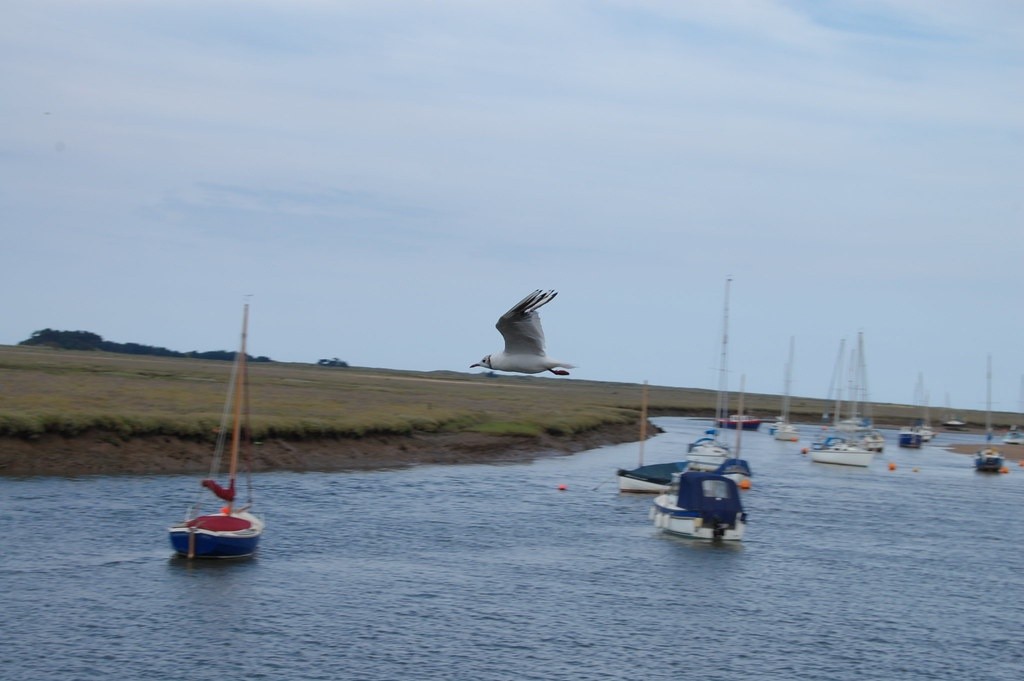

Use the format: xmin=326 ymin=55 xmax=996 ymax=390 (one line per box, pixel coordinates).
xmin=714 ymin=411 xmax=762 ymax=432
xmin=1003 ymin=430 xmax=1024 ymax=446
xmin=942 ymin=419 xmax=967 ymax=430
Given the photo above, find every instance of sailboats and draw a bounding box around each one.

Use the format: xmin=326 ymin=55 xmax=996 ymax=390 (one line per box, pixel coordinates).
xmin=771 ymin=335 xmax=935 ymax=467
xmin=167 ymin=301 xmax=264 ymax=561
xmin=973 ymin=355 xmax=1006 ymax=472
xmin=615 ymin=277 xmax=752 ymax=541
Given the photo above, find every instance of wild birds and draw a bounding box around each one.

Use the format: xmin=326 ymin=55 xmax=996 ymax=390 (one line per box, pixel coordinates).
xmin=469 ymin=289 xmax=577 ymax=375
